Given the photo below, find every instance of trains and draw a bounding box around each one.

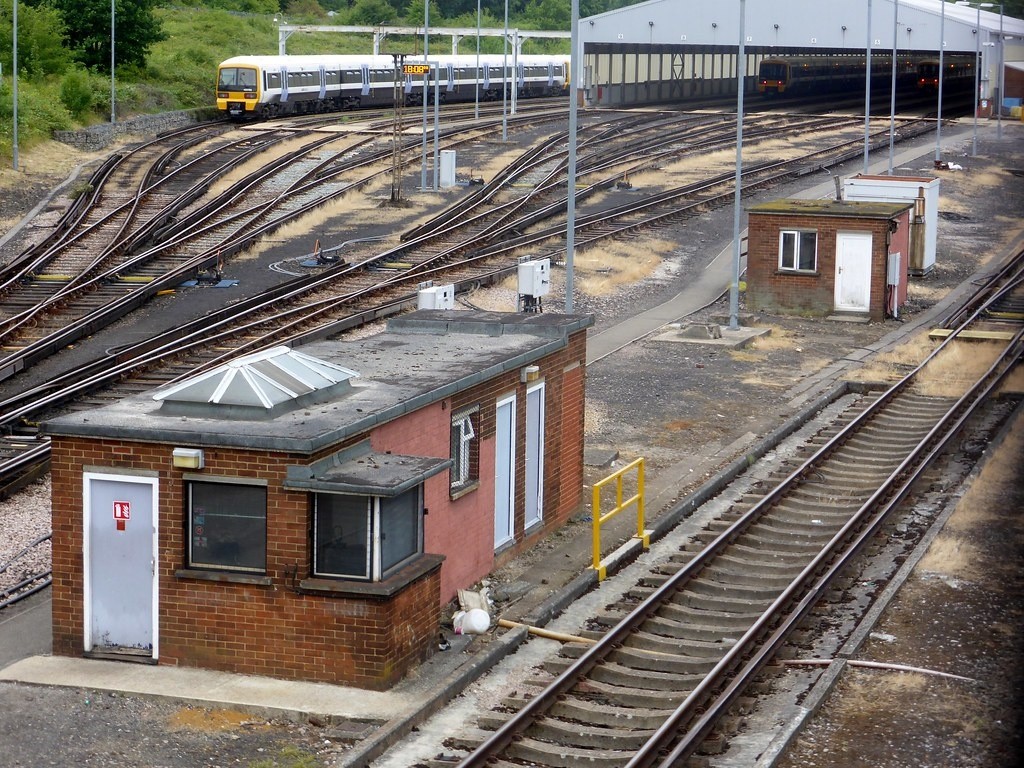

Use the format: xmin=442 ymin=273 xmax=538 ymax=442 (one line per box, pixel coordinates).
xmin=756 ymin=55 xmax=924 ymax=102
xmin=217 ymin=53 xmax=573 ymax=121
xmin=917 ymin=55 xmax=981 ymax=93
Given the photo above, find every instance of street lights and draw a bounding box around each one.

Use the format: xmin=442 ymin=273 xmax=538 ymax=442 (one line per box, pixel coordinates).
xmin=979 ymin=3 xmax=1004 ymax=134
xmin=954 ymin=1 xmax=982 ymax=156
